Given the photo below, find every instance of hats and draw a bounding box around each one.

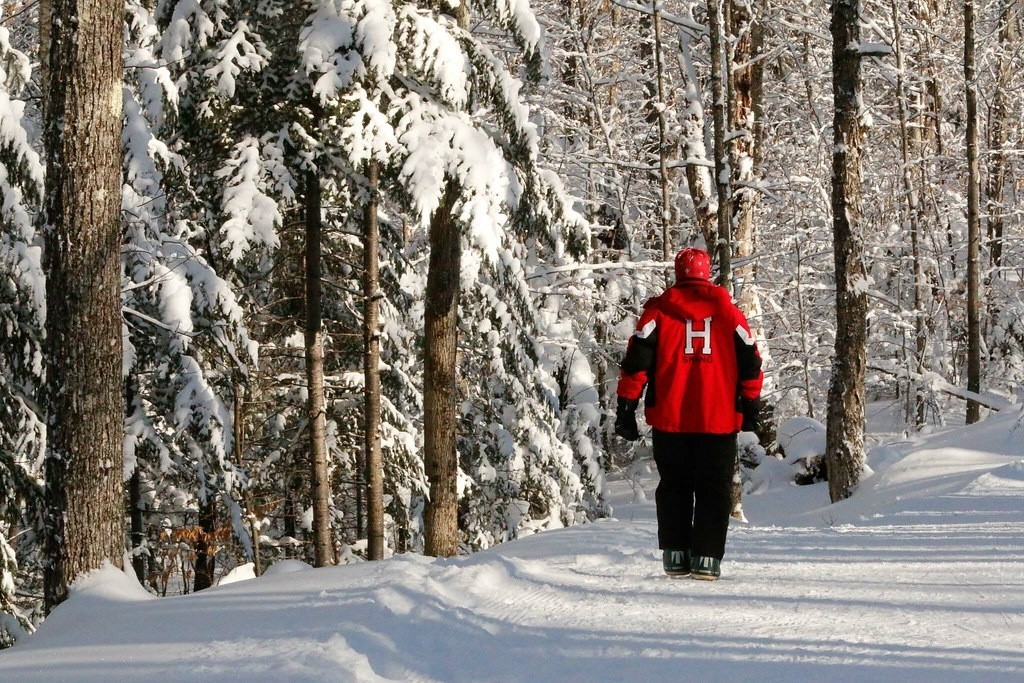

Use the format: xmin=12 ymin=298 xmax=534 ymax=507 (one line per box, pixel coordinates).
xmin=674 ymin=247 xmax=712 ymax=281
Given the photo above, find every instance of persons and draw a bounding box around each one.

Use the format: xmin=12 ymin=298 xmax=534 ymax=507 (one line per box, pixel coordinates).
xmin=614 ymin=247 xmax=764 ymax=578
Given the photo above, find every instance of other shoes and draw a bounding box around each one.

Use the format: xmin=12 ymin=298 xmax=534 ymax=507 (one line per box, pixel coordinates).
xmin=689 ymin=556 xmax=721 ymax=580
xmin=662 ymin=549 xmax=692 ymax=578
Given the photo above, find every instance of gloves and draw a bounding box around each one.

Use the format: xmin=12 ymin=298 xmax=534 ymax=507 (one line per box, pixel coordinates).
xmin=614 ymin=396 xmax=640 ymax=441
xmin=735 ymin=392 xmax=762 ymax=432
